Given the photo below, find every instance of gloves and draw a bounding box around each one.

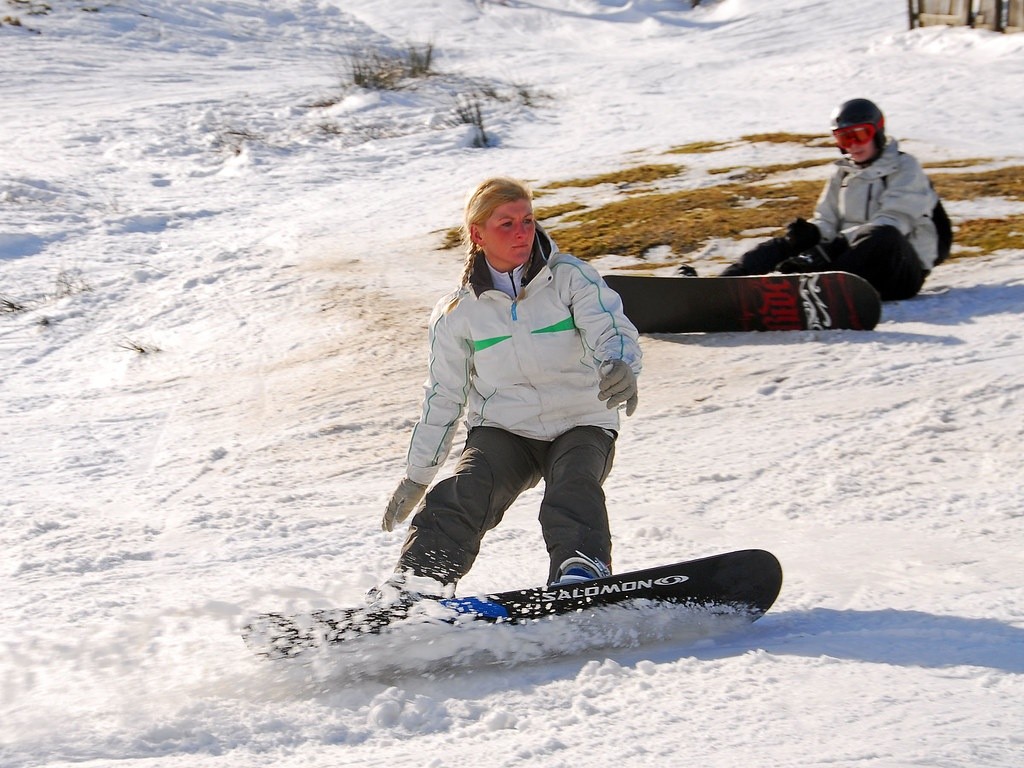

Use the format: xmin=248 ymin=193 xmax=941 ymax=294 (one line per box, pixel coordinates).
xmin=598 ymin=359 xmax=638 ymax=416
xmin=785 ymin=217 xmax=820 ymax=252
xmin=812 ymin=233 xmax=850 ymax=267
xmin=382 ymin=477 xmax=428 ymax=532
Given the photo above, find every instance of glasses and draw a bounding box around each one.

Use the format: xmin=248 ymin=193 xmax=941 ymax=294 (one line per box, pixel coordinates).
xmin=831 ymin=121 xmax=877 ymax=149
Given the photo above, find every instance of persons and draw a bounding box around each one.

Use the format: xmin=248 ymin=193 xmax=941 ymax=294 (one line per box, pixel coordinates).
xmin=379 ymin=179 xmax=643 ymax=588
xmin=676 ymin=98 xmax=954 ymax=299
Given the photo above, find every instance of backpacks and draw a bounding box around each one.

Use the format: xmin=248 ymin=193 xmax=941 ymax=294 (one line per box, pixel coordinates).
xmin=841 ymin=151 xmax=953 ymax=266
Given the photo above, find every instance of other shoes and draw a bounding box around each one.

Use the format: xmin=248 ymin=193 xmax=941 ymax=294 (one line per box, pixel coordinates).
xmin=366 ymin=585 xmax=415 ymax=603
xmin=561 ymin=563 xmax=598 ymax=584
xmin=677 ymin=266 xmax=698 ymax=276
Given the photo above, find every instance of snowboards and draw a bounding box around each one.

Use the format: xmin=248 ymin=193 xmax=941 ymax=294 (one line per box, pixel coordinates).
xmin=605 ymin=269 xmax=884 ymax=334
xmin=242 ymin=546 xmax=784 ymax=667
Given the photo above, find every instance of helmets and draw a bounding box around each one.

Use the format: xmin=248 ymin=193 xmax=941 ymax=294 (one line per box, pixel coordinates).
xmin=832 ymin=99 xmax=886 ymax=154
